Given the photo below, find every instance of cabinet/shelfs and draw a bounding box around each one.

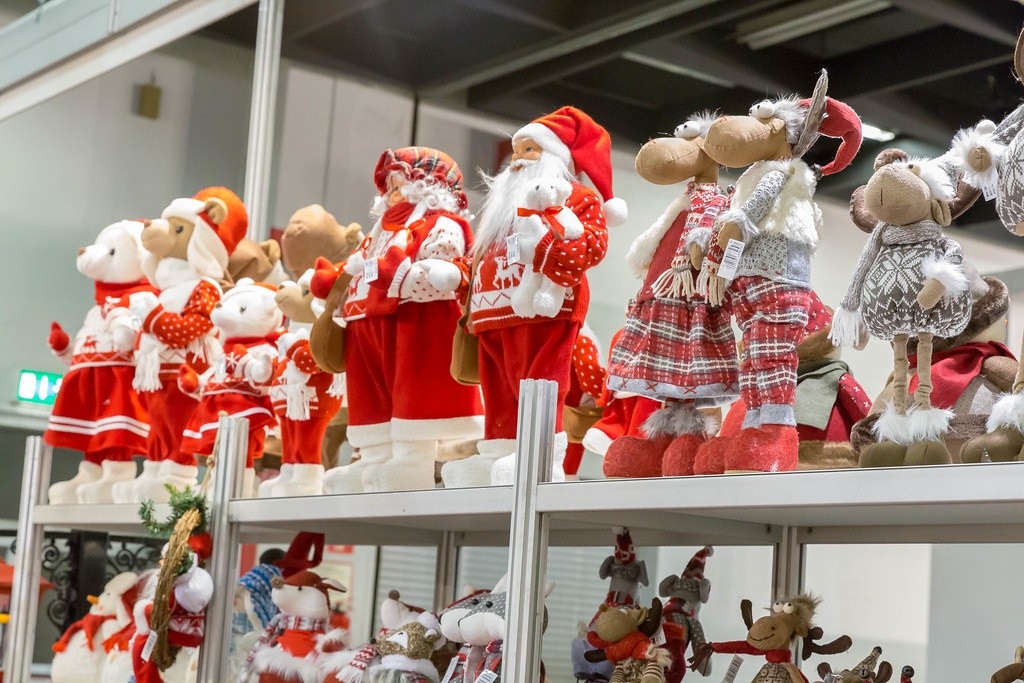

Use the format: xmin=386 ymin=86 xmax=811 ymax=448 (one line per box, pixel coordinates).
xmin=2 ymin=378 xmax=1024 ymax=683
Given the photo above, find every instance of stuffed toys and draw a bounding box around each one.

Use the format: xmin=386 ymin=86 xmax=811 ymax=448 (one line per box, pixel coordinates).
xmin=42 ymin=182 xmax=366 ymax=509
xmin=600 ymin=26 xmax=1024 ymax=480
xmin=50 ymin=532 xmax=558 ymax=683
xmin=571 ymin=524 xmax=1024 ymax=683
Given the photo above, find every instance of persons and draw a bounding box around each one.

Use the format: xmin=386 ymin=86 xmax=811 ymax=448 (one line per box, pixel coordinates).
xmin=308 ymin=145 xmax=487 ymax=495
xmin=418 ymin=103 xmax=628 ymax=489
